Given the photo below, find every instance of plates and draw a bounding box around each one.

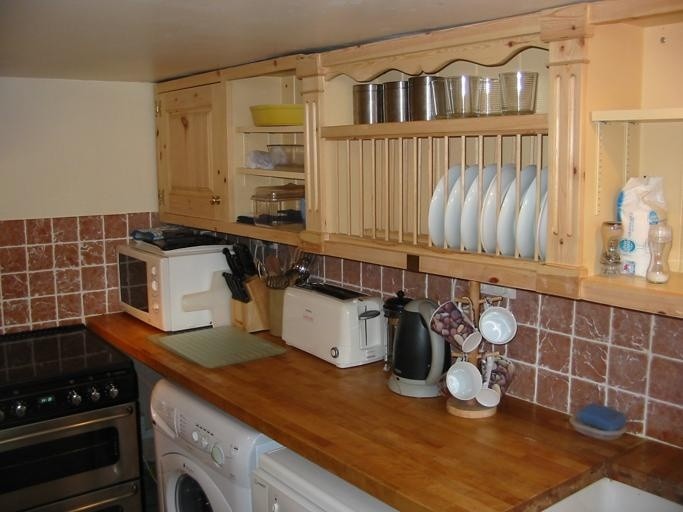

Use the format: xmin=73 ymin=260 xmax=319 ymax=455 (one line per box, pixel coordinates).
xmin=428 ymin=164 xmax=549 ymax=262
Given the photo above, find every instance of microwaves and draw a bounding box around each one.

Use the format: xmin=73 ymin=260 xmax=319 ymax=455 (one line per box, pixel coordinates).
xmin=115 ymin=244 xmax=235 ymax=331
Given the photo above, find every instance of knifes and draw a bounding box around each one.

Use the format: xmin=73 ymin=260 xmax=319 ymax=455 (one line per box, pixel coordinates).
xmin=222 ymin=242 xmax=259 ymax=303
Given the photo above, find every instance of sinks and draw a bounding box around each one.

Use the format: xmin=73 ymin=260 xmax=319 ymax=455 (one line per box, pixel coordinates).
xmin=503 ymin=458 xmax=683 ymax=512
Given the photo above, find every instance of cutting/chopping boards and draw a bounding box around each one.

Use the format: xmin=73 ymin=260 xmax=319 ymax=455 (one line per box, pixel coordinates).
xmin=182 ymin=269 xmax=233 ymax=329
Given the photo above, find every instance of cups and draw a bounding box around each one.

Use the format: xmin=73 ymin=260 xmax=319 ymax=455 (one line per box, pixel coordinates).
xmin=431 ymin=71 xmax=539 ymax=120
xmin=383 ymin=290 xmax=414 ymax=372
xmin=429 ymin=296 xmax=482 ymax=353
xmin=478 ymin=297 xmax=517 ymax=345
xmin=436 ymin=357 xmax=482 ymax=400
xmin=475 ymin=353 xmax=516 ymax=407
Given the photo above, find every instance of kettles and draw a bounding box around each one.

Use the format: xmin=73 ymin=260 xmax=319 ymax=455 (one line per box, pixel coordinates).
xmin=388 ymin=298 xmax=452 ymax=399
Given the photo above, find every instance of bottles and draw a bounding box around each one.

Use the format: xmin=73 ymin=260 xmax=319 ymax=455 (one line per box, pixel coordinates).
xmin=645 ymin=224 xmax=673 ymax=285
xmin=600 ymin=220 xmax=623 ymax=274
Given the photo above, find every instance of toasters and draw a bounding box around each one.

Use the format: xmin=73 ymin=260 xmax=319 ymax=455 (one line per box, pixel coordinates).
xmin=282 ymin=282 xmax=388 ymax=369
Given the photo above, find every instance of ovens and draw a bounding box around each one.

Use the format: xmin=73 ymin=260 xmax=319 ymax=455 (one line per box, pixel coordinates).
xmin=0 ymin=324 xmax=144 ymax=512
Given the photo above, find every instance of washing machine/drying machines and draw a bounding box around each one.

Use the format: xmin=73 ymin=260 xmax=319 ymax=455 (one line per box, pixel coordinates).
xmin=252 ymin=446 xmax=402 ymax=512
xmin=148 ymin=378 xmax=281 ymax=512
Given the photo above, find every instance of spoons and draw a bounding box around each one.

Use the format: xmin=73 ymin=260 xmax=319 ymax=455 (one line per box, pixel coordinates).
xmin=256 ymin=260 xmax=269 ymax=288
xmin=295 ymin=257 xmax=308 ymax=280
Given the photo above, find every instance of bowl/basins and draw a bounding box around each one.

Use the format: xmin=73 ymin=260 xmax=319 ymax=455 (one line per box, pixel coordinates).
xmin=267 ymin=144 xmax=305 ymax=169
xmin=249 ymin=103 xmax=305 ymax=127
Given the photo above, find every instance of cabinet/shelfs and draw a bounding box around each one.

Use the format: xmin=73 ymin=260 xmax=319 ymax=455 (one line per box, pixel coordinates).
xmin=154 ymin=1 xmax=683 ymax=320
xmin=88 ymin=290 xmax=683 ymax=512
